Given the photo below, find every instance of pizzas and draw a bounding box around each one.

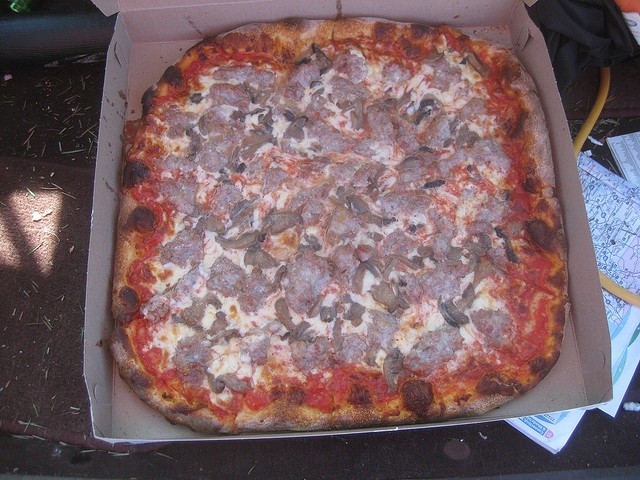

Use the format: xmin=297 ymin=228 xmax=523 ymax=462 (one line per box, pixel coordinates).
xmin=111 ymin=17 xmax=568 ymax=433
